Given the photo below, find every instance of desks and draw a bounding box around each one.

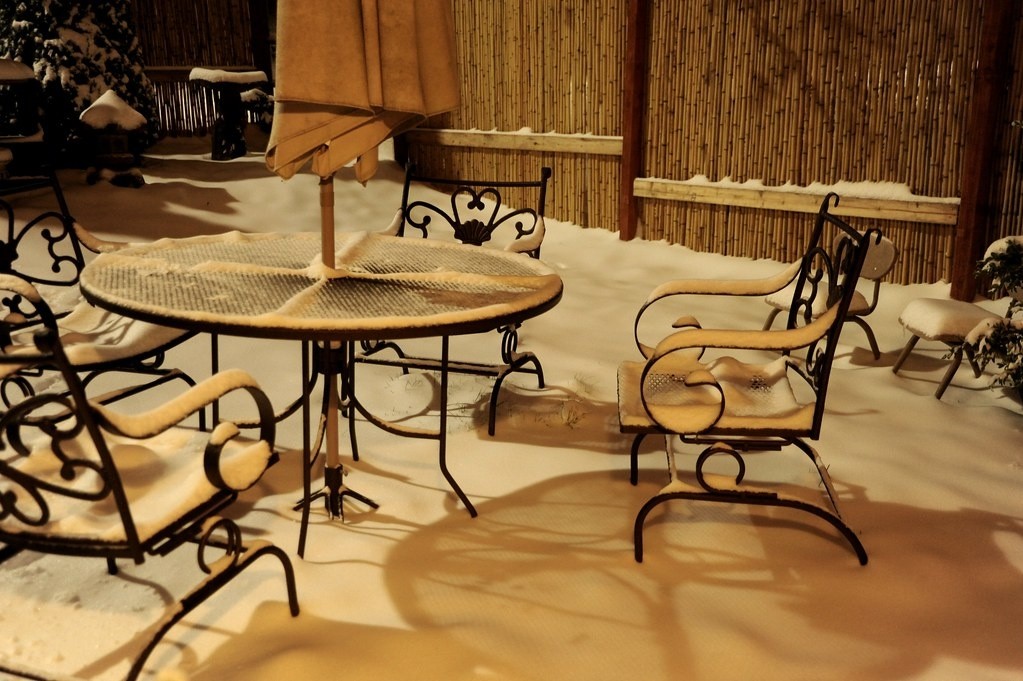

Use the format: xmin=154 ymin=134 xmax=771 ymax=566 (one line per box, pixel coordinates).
xmin=82 ymin=229 xmax=563 ymax=522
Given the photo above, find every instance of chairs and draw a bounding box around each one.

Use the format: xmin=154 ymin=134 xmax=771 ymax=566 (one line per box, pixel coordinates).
xmin=893 ymin=234 xmax=1022 ymax=399
xmin=346 ymin=162 xmax=552 ymax=518
xmin=764 ymin=230 xmax=894 ymax=359
xmin=0 ymin=175 xmax=219 ymax=432
xmin=617 ymin=190 xmax=883 ymax=566
xmin=0 ymin=270 xmax=299 ymax=680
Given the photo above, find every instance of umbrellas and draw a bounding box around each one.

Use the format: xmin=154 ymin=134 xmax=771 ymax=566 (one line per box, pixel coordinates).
xmin=262 ymin=0 xmax=457 ymax=527
